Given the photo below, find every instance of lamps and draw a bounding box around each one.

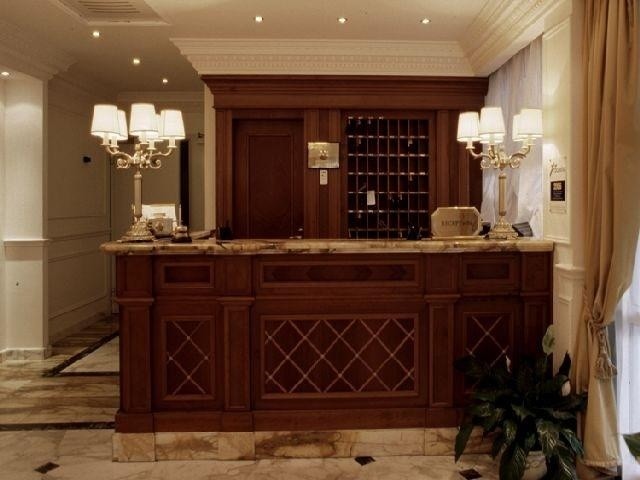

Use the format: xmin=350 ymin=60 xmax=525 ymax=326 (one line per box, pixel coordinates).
xmin=91 ymin=101 xmax=187 ymax=243
xmin=457 ymin=106 xmax=543 ymax=238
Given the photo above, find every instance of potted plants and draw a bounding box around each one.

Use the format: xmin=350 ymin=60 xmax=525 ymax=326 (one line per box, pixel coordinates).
xmin=454 ymin=354 xmax=588 ymax=480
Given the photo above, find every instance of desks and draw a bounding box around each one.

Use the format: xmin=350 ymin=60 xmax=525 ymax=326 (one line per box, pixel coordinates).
xmin=100 ymin=232 xmax=555 ymax=433
